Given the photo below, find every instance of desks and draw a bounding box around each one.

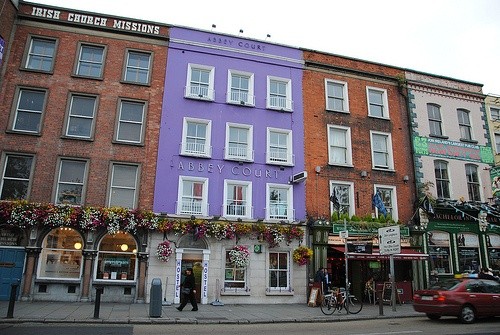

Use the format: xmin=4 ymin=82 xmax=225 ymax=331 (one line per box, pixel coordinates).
xmin=376 ymin=282 xmax=412 ymax=303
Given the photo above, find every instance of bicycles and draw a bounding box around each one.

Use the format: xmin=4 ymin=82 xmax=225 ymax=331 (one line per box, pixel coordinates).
xmin=320 ymin=282 xmax=363 ymax=315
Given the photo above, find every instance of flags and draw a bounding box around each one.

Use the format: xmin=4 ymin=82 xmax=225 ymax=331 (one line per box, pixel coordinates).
xmin=420 ymin=196 xmax=435 ymax=218
xmin=329 ymin=187 xmax=340 ymax=209
xmin=373 ymin=192 xmax=386 ymax=218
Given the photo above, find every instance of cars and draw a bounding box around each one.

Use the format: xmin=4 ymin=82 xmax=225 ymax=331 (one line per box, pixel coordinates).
xmin=410 ymin=277 xmax=500 ymax=324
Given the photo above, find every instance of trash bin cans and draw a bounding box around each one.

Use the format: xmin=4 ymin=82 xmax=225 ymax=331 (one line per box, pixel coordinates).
xmin=148 ymin=277 xmax=163 ymax=318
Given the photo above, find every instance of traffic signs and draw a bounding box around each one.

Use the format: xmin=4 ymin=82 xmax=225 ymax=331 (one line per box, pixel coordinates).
xmin=378 ymin=225 xmax=401 ymax=255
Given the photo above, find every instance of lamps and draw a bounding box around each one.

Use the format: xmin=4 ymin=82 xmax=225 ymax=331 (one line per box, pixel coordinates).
xmin=361 ymin=171 xmax=367 ymax=181
xmin=403 ymin=176 xmax=409 ymax=184
xmin=315 ymin=166 xmax=321 ymax=175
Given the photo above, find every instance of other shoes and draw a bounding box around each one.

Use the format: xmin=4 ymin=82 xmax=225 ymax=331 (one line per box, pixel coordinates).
xmin=176 ymin=307 xmax=182 ymax=311
xmin=191 ymin=308 xmax=198 ymax=311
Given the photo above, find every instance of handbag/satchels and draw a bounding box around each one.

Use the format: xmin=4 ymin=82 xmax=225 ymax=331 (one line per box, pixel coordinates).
xmin=182 ymin=287 xmax=190 ymax=294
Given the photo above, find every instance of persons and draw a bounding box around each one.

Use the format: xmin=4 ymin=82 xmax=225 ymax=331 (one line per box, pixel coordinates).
xmin=365 ymin=276 xmax=377 ymax=302
xmin=317 ymin=267 xmax=331 ymax=305
xmin=176 ymin=268 xmax=198 ymax=311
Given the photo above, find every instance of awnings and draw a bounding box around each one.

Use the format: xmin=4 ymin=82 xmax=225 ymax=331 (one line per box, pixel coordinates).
xmin=331 ymin=246 xmax=429 ymax=260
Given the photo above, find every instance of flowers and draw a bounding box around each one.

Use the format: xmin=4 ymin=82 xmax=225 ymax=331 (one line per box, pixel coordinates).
xmin=0 ymin=199 xmax=313 ymax=268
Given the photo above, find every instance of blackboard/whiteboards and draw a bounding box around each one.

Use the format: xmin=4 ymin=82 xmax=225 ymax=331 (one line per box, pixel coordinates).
xmin=382 ymin=282 xmax=400 ymax=302
